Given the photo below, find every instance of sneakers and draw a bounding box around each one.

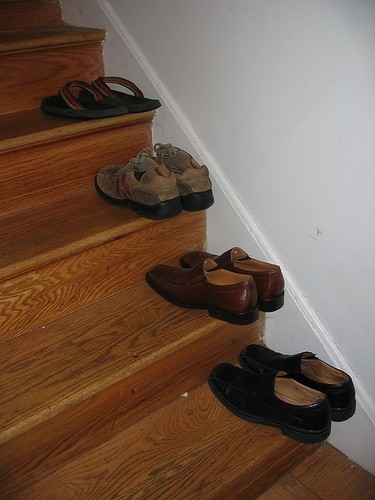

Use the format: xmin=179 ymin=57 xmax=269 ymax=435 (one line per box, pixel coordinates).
xmin=149 ymin=143 xmax=215 ymax=213
xmin=95 ymin=149 xmax=183 ymax=220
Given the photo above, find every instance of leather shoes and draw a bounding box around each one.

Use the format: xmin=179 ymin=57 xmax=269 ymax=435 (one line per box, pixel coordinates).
xmin=237 ymin=344 xmax=357 ymax=423
xmin=208 ymin=361 xmax=332 ymax=444
xmin=145 ymin=259 xmax=260 ymax=326
xmin=179 ymin=247 xmax=285 ymax=313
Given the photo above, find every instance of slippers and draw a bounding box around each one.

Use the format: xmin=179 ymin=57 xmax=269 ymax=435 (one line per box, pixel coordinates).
xmin=39 ymin=80 xmax=129 ymax=119
xmin=80 ymin=76 xmax=161 ymax=113
